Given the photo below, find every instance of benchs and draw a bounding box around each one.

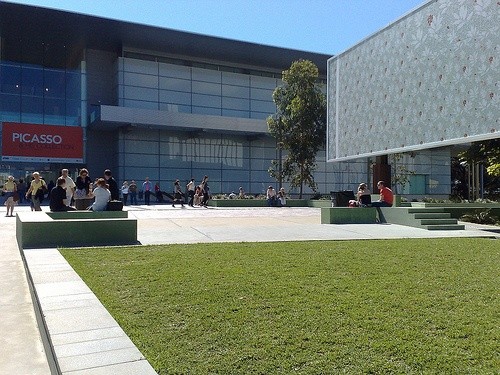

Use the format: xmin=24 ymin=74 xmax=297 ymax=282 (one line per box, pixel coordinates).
xmin=15 ymin=209 xmax=138 ymax=249
xmin=320 ymin=207 xmax=377 ymax=224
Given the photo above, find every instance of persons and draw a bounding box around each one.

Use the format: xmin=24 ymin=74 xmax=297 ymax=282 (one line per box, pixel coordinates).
xmin=103 ymin=169 xmax=120 ymax=201
xmin=155 ymin=182 xmax=160 ymax=202
xmin=49 ymin=179 xmax=76 ymax=211
xmin=88 ymin=178 xmax=111 ymax=211
xmin=142 ymin=177 xmax=153 ymax=205
xmin=349 ymin=183 xmax=372 ymax=207
xmin=120 ymin=180 xmax=137 ymax=206
xmin=239 ymin=187 xmax=245 ymax=197
xmin=266 ymin=186 xmax=287 ymax=206
xmin=56 ymin=168 xmax=76 ymax=207
xmin=75 ymin=168 xmax=91 ymax=196
xmin=172 ymin=175 xmax=209 ymax=207
xmin=2 ymin=171 xmax=47 ymax=217
xmin=229 ymin=191 xmax=236 ymax=199
xmin=371 ymin=181 xmax=393 ymax=208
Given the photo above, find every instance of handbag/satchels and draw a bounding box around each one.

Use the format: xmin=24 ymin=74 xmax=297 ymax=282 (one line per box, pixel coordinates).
xmin=348 ymin=202 xmax=357 ymax=207
xmin=41 ymin=180 xmax=47 ymax=193
